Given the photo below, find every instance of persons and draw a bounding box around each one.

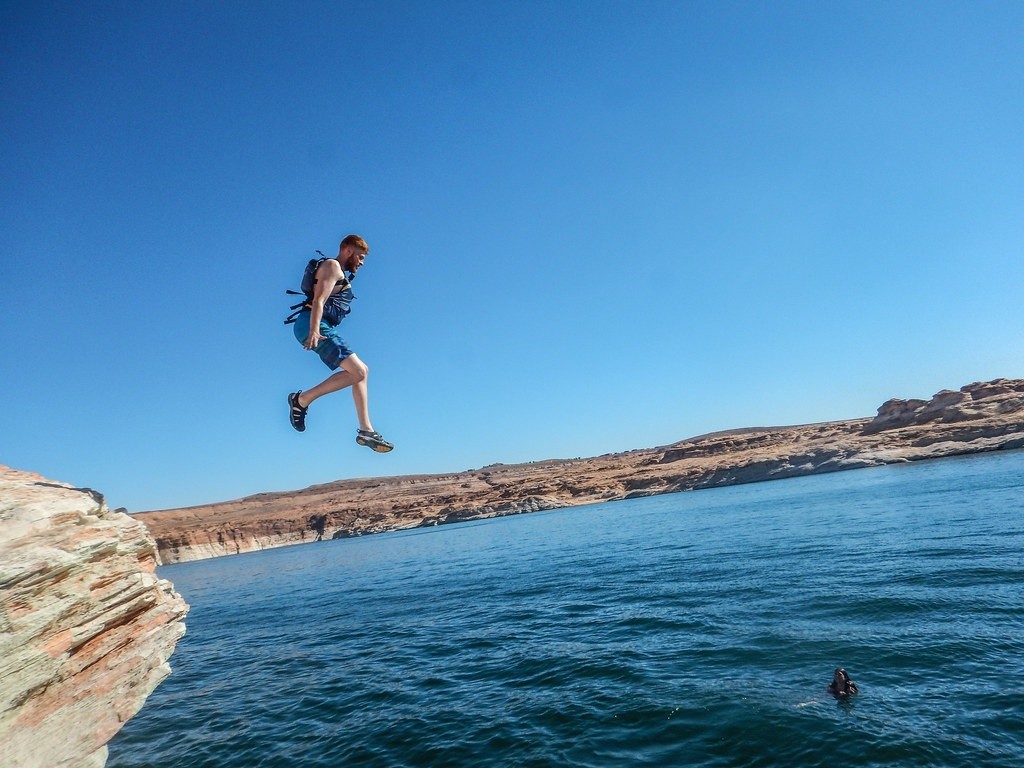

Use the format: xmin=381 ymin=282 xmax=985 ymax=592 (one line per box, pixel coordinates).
xmin=831 ymin=668 xmax=857 ymax=694
xmin=287 ymin=234 xmax=394 ymax=454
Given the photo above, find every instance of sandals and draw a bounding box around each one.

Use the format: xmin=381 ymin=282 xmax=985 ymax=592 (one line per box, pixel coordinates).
xmin=288 ymin=390 xmax=308 ymax=432
xmin=356 ymin=429 xmax=394 ymax=453
